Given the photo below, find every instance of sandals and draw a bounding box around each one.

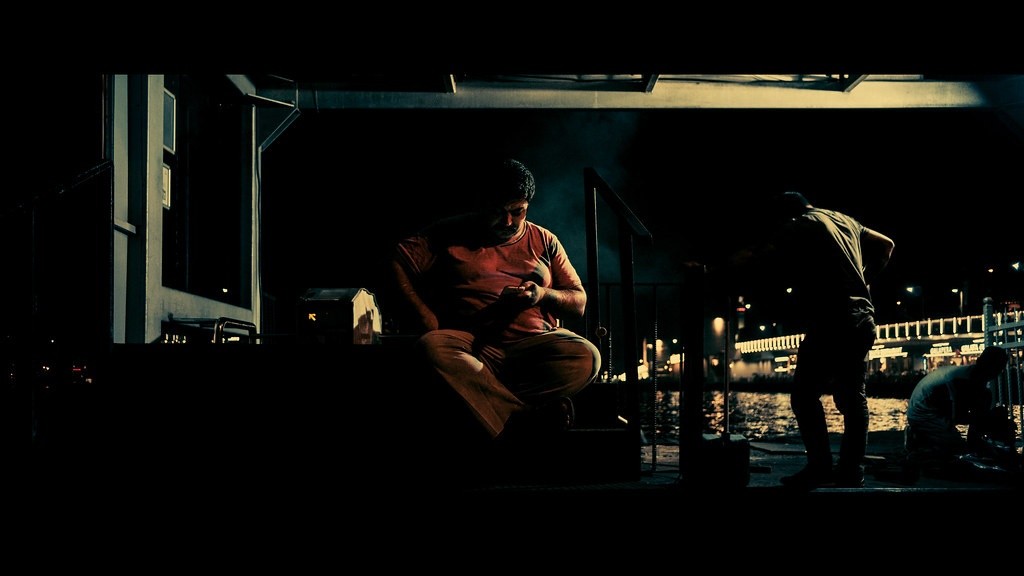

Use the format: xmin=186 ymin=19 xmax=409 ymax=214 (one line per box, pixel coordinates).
xmin=530 ymin=393 xmax=576 ymax=431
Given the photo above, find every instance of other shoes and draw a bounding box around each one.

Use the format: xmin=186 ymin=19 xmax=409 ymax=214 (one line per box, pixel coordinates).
xmin=780 ymin=465 xmax=827 ymax=487
xmin=845 ymin=468 xmax=865 ymax=488
xmin=904 ymin=426 xmax=917 ymax=453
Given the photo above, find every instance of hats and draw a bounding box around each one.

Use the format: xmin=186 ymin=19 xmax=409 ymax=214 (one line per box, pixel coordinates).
xmin=979 ymin=346 xmax=1008 ymax=371
xmin=773 ymin=192 xmax=813 ymax=222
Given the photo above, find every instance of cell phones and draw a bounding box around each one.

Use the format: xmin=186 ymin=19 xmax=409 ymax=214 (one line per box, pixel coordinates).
xmin=498 ymin=286 xmax=525 ymax=299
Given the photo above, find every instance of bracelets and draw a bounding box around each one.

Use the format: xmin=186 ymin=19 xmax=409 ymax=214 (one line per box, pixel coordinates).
xmin=704 ymin=264 xmax=707 ymax=273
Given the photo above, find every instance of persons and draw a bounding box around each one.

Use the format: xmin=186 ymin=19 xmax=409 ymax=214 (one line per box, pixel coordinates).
xmin=681 ymin=191 xmax=895 ymax=489
xmin=903 ymin=347 xmax=1017 ymax=461
xmin=389 ymin=160 xmax=602 ymax=439
